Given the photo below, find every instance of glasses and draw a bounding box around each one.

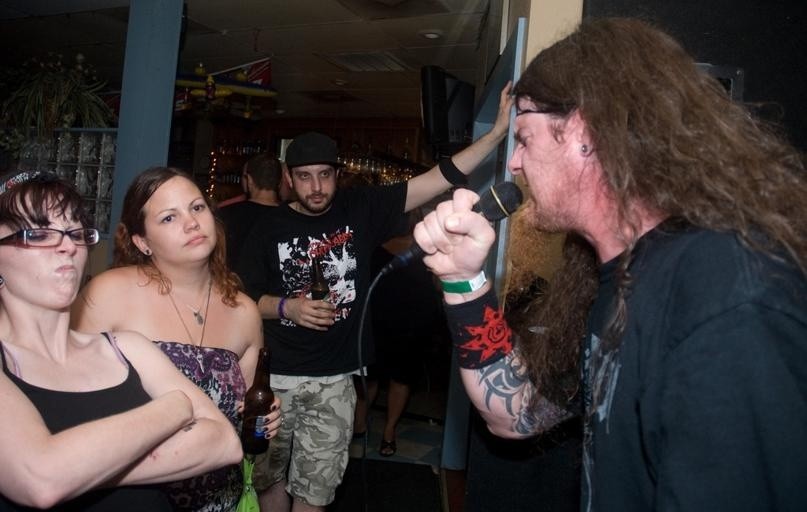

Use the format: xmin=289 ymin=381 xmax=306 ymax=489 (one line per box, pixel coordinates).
xmin=1 ymin=228 xmax=100 ymax=249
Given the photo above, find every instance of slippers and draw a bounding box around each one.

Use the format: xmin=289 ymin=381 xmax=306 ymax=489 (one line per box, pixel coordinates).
xmin=379 ymin=434 xmax=397 ymax=456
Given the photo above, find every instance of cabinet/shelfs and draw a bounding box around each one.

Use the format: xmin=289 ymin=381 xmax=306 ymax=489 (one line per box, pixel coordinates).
xmin=21 ymin=125 xmax=118 ymax=239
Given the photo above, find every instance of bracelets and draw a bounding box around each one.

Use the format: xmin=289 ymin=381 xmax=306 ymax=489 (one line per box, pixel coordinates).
xmin=440 ymin=155 xmax=467 ymax=186
xmin=439 ymin=270 xmax=488 ymax=294
xmin=437 ymin=288 xmax=518 ymax=370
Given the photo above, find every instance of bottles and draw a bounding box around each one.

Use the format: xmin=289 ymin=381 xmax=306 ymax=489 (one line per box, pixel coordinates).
xmin=241 ymin=345 xmax=275 ymax=455
xmin=208 ymin=132 xmax=424 ymax=200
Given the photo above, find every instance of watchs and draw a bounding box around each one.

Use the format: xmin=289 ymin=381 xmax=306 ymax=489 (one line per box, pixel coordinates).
xmin=278 ymin=297 xmax=296 ymax=328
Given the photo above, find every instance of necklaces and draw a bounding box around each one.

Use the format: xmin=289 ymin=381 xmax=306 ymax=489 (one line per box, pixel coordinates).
xmin=164 ymin=273 xmax=210 ymax=326
xmin=166 ymin=287 xmax=211 ymax=371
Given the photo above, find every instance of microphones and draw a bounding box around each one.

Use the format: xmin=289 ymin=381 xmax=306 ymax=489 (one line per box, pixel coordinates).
xmin=408 ymin=182 xmax=522 ymax=262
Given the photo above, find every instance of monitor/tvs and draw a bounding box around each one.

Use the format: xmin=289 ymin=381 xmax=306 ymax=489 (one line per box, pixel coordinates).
xmin=417 ymin=65 xmax=475 ymax=164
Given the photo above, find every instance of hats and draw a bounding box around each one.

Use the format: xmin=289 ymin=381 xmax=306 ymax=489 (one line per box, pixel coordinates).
xmin=285 ymin=132 xmax=348 ymax=170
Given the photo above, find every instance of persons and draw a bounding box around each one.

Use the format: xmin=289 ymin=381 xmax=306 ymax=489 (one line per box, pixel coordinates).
xmin=71 ymin=167 xmax=266 ymax=511
xmin=0 ymin=168 xmax=245 ymax=511
xmin=214 ymin=152 xmax=284 ymax=304
xmin=225 ymin=78 xmax=518 ymax=512
xmin=353 ymin=233 xmax=418 ymax=461
xmin=410 ymin=20 xmax=806 ymax=510
